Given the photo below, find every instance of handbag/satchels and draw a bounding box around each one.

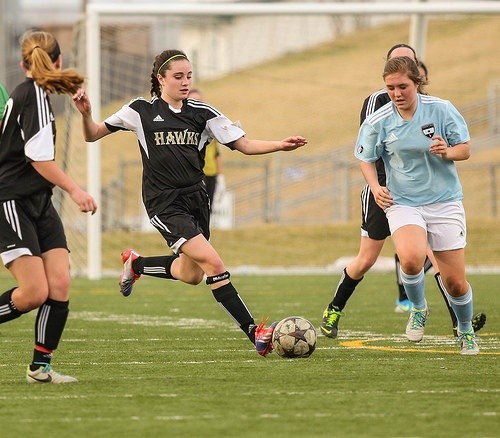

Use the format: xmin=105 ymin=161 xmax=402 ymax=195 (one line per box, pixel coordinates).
xmin=210 ymin=174 xmax=234 ymax=230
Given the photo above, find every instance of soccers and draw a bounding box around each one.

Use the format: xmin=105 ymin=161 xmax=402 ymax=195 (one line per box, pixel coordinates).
xmin=272 ymin=316 xmax=317 ymax=358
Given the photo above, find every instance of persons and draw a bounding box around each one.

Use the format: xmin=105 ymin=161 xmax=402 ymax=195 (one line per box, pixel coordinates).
xmin=70 ymin=50 xmax=308 ymax=356
xmin=187 ymin=87 xmax=220 ymax=211
xmin=0 ymin=30 xmax=98 ymax=384
xmin=321 ymin=44 xmax=487 ymax=338
xmin=354 ymin=56 xmax=479 ymax=356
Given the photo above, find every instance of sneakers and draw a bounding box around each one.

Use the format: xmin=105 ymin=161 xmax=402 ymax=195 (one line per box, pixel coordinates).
xmin=254 ymin=318 xmax=279 ymax=356
xmin=321 ymin=303 xmax=345 ymax=339
xmin=26 ymin=362 xmax=79 ymax=384
xmin=395 ymin=299 xmax=429 ymax=313
xmin=452 ymin=312 xmax=485 ymax=336
xmin=405 ymin=305 xmax=427 ymax=342
xmin=118 ymin=248 xmax=141 ymax=298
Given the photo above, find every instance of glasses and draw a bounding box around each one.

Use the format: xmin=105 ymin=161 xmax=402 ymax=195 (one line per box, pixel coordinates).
xmin=455 ymin=327 xmax=479 ymax=356
